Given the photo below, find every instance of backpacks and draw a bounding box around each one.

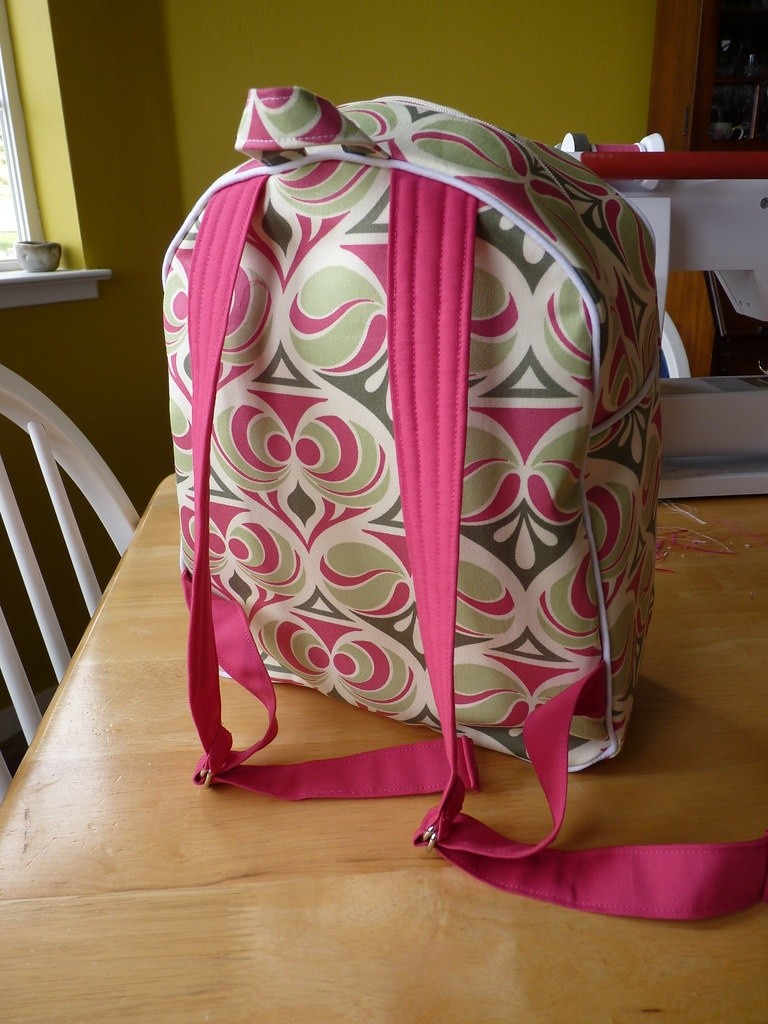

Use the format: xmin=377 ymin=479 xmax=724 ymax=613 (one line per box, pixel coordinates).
xmin=162 ymin=85 xmax=768 ymax=920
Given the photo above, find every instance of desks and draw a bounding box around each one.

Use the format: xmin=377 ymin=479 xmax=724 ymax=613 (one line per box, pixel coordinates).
xmin=1 ymin=474 xmax=767 ymax=1024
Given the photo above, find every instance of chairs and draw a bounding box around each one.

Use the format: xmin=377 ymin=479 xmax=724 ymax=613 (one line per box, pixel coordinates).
xmin=1 ymin=363 xmax=142 ymax=802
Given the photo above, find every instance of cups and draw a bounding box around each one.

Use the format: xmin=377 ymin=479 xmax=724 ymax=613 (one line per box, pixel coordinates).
xmin=710 ymin=122 xmax=745 ymax=140
xmin=718 ymin=34 xmax=768 ymax=78
